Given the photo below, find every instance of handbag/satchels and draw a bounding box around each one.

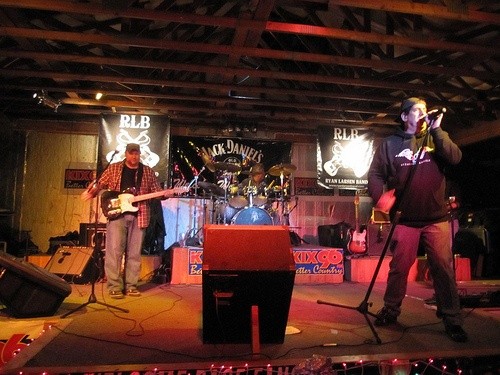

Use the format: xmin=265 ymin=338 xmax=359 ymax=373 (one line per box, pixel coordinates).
xmin=452 ymin=224 xmax=491 ymax=258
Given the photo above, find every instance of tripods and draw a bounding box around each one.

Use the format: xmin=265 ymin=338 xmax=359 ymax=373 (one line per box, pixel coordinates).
xmin=57 ymin=154 xmax=129 ymax=319
xmin=182 ymin=171 xmax=311 ymax=247
xmin=316 ymin=120 xmax=434 ymax=345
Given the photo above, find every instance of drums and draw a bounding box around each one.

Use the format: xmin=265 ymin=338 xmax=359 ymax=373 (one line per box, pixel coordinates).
xmin=230 ymin=206 xmax=275 ymax=226
xmin=225 ymin=194 xmax=267 ymax=221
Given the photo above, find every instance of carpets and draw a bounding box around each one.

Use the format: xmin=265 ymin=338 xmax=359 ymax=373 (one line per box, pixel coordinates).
xmin=6 ymin=279 xmax=499 ymax=375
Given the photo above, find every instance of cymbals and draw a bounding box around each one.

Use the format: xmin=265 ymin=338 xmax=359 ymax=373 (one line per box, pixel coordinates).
xmin=198 ymin=182 xmax=226 ymax=197
xmin=214 ymin=162 xmax=241 ymax=173
xmin=268 ymin=164 xmax=297 ymax=177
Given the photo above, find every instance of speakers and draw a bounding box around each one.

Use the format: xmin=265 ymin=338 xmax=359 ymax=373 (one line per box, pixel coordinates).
xmin=47 ymin=245 xmax=100 ymax=284
xmin=0 ymin=250 xmax=72 ymax=318
xmin=79 ymin=223 xmax=107 ymax=255
xmin=366 ymin=222 xmax=395 ymax=256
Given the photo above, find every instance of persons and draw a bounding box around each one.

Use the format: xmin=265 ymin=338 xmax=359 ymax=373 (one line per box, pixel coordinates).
xmin=81 ymin=143 xmax=176 ymax=298
xmin=238 ymin=164 xmax=269 ymax=196
xmin=368 ymin=97 xmax=468 ymax=342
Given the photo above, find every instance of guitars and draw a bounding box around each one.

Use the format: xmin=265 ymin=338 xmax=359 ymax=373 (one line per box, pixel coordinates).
xmin=347 ymin=191 xmax=368 ymax=254
xmin=101 ymin=186 xmax=189 ymax=220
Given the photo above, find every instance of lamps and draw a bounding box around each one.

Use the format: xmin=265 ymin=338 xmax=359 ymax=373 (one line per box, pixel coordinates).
xmin=34 ymin=92 xmax=62 ymax=112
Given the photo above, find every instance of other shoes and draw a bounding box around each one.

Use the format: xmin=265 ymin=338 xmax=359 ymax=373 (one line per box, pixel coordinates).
xmin=374 ymin=307 xmax=396 ymax=325
xmin=445 ymin=325 xmax=468 ymax=342
xmin=109 ymin=290 xmax=124 ymax=299
xmin=124 ymin=289 xmax=142 ymax=296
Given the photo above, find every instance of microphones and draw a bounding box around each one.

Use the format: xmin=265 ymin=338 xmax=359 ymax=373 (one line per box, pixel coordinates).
xmin=111 ymin=149 xmax=120 ymax=158
xmin=423 ymin=106 xmax=446 ymax=121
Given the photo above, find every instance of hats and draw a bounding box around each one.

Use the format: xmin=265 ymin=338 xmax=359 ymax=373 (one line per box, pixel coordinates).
xmin=394 ymin=98 xmax=427 ymax=122
xmin=126 ymin=143 xmax=139 ymax=153
